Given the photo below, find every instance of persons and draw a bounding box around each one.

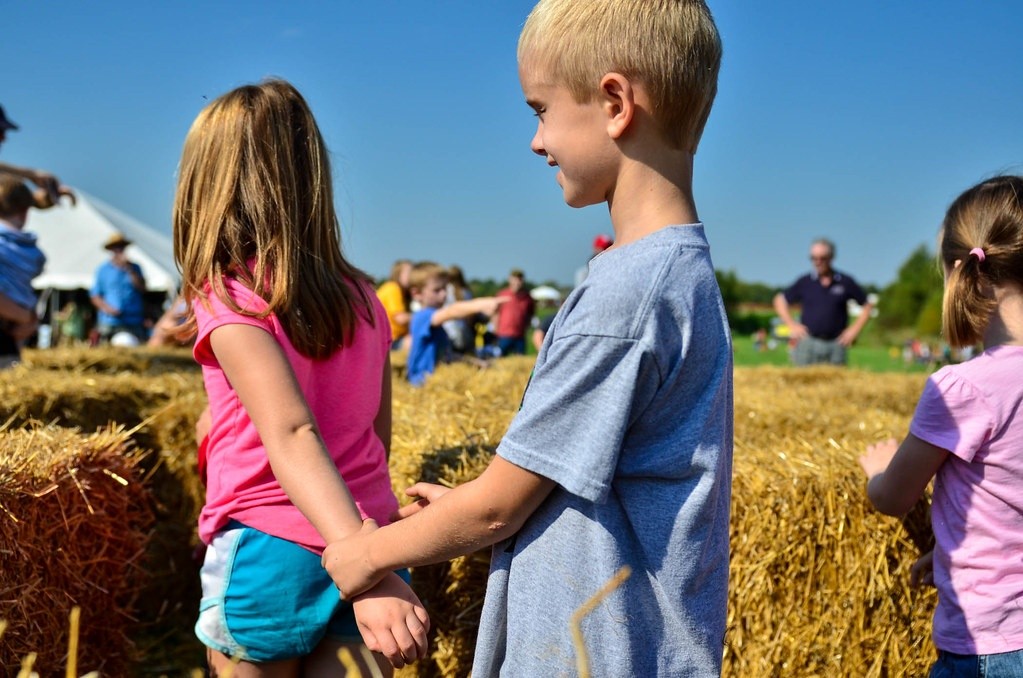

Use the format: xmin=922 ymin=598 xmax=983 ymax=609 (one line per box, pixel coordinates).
xmin=376 ymin=252 xmax=564 ymax=377
xmin=158 ymin=79 xmax=433 ymax=678
xmin=771 ymin=240 xmax=876 ymax=365
xmin=320 ymin=0 xmax=734 ymax=678
xmin=856 ymin=174 xmax=1023 ymax=677
xmin=0 ymin=108 xmax=82 ymax=365
xmin=89 ymin=230 xmax=150 ymax=342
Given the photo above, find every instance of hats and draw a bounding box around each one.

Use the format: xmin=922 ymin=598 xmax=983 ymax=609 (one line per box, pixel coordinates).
xmin=103 ymin=236 xmax=131 ymax=252
